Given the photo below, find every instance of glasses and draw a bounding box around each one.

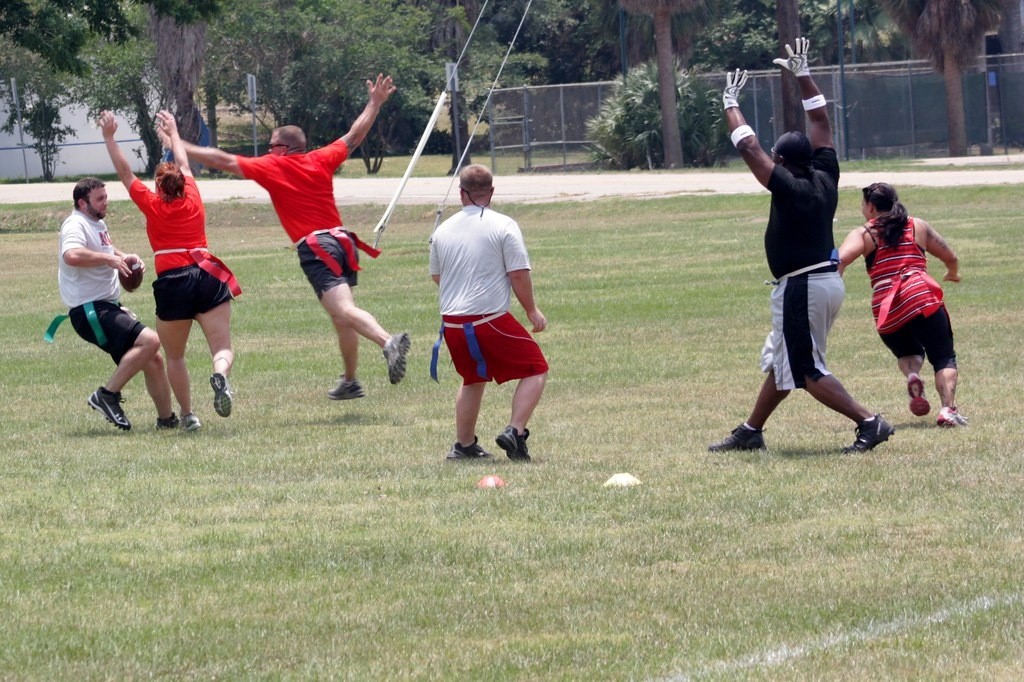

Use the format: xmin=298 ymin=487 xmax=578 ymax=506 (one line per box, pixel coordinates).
xmin=268 ymin=142 xmax=289 ymax=150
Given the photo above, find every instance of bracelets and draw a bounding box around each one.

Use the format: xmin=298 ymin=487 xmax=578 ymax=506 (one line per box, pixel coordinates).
xmin=730 ymin=124 xmax=756 ymax=148
xmin=801 ymin=94 xmax=827 ymax=111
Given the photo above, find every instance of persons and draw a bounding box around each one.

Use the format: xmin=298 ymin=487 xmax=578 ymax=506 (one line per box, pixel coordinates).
xmin=428 ymin=164 xmax=550 ymax=463
xmin=45 ymin=176 xmax=182 ymax=432
xmin=707 ymin=36 xmax=896 ymax=454
xmin=158 ymin=72 xmax=411 ymax=401
xmin=837 ymin=182 xmax=968 ymax=426
xmin=97 ymin=109 xmax=244 ymax=433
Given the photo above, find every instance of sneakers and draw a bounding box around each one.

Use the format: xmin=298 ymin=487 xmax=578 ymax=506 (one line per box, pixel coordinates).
xmin=495 ymin=423 xmax=530 ymax=463
xmin=908 ymin=374 xmax=930 ymax=417
xmin=155 ymin=412 xmax=181 ymax=429
xmin=210 ymin=371 xmax=232 ymax=418
xmin=182 ymin=412 xmax=202 ymax=430
xmin=88 ymin=387 xmax=131 ymax=432
xmin=327 ymin=376 xmax=363 ymax=400
xmin=707 ymin=423 xmax=767 ymax=455
xmin=384 ymin=332 xmax=409 ymax=384
xmin=446 ymin=436 xmax=495 ymax=461
xmin=937 ymin=406 xmax=969 ymax=429
xmin=842 ymin=412 xmax=895 ymax=457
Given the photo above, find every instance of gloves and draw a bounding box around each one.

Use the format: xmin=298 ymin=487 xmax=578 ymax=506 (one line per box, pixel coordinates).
xmin=773 ymin=37 xmax=810 ymax=77
xmin=723 ymin=68 xmax=749 ymax=110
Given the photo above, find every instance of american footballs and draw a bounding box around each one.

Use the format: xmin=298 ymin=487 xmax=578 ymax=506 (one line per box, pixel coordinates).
xmin=477 ymin=474 xmax=506 ymax=488
xmin=119 ymin=257 xmax=145 ymax=292
